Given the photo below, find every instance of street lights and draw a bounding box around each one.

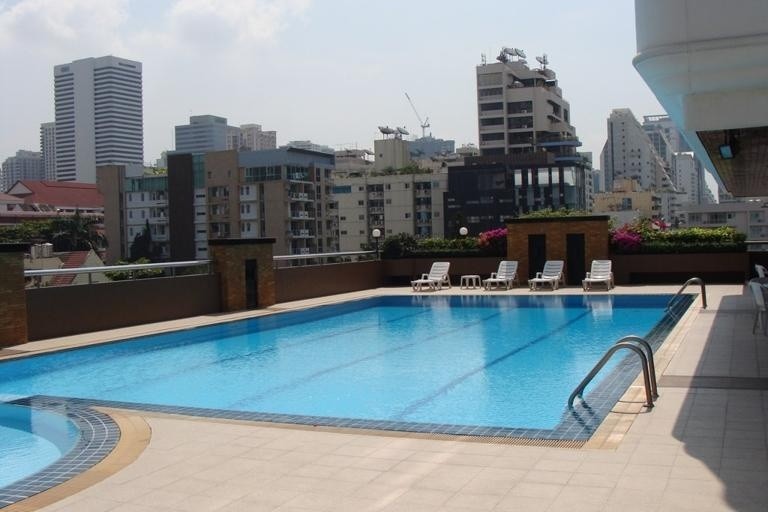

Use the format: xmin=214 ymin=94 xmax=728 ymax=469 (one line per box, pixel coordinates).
xmin=458 ymin=226 xmax=469 ymax=239
xmin=372 ymin=228 xmax=383 ymax=261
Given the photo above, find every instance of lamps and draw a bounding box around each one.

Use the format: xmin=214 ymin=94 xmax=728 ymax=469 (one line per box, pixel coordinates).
xmin=459 ymin=227 xmax=468 ymax=239
xmin=719 ymin=130 xmax=741 ymax=160
xmin=372 ymin=229 xmax=381 ymax=249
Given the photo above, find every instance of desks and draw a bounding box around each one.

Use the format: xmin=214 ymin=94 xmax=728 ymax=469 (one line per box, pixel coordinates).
xmin=461 ymin=275 xmax=481 ymax=289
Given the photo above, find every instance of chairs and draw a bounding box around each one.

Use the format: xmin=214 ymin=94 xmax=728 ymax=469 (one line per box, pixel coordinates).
xmin=749 ymin=282 xmax=768 ymax=336
xmin=755 ymin=264 xmax=768 ymax=278
xmin=581 ymin=259 xmax=615 ymax=291
xmin=583 ymin=294 xmax=614 ymax=321
xmin=482 ymin=260 xmax=521 ymax=290
xmin=527 ymin=260 xmax=567 ymax=291
xmin=410 ymin=262 xmax=452 ymax=291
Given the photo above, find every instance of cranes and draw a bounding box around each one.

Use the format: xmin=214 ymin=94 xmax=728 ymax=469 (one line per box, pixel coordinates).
xmin=404 ymin=91 xmax=431 ymax=138
